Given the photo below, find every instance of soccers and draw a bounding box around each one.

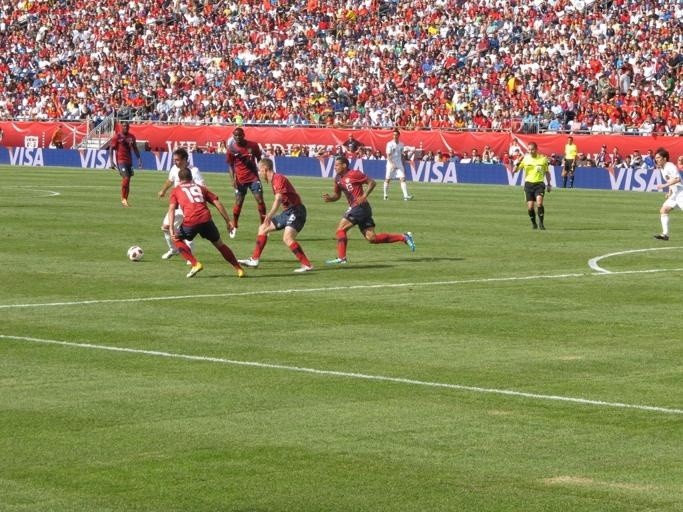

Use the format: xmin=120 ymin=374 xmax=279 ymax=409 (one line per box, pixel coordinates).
xmin=127 ymin=246 xmax=144 ymax=261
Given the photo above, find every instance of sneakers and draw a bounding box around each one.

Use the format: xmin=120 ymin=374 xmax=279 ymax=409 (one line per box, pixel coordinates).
xmin=654 ymin=233 xmax=669 ymax=241
xmin=326 ymin=258 xmax=347 ymax=265
xmin=404 ymin=232 xmax=416 ymax=252
xmin=187 ymin=263 xmax=203 ymax=278
xmin=384 ymin=196 xmax=388 ymax=199
xmin=532 ymin=225 xmax=544 ymax=229
xmin=162 ymin=249 xmax=179 ymax=259
xmin=239 ymin=259 xmax=260 ymax=267
xmin=238 ymin=270 xmax=248 ymax=277
xmin=230 ymin=228 xmax=237 ymax=239
xmin=121 ymin=199 xmax=130 ymax=207
xmin=405 ymin=196 xmax=413 ymax=201
xmin=294 ymin=266 xmax=312 ymax=273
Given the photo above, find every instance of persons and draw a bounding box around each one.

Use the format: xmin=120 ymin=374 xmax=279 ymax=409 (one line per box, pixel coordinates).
xmin=656 ymin=147 xmax=683 ymax=239
xmin=169 ymin=168 xmax=246 ymax=277
xmin=160 ymin=149 xmax=205 ymax=259
xmin=0 ymin=0 xmax=683 ymax=170
xmin=238 ymin=158 xmax=314 ymax=272
xmin=512 ymin=142 xmax=550 ymax=230
xmin=321 ymin=157 xmax=415 ymax=264
xmin=110 ymin=122 xmax=141 ymax=206
xmin=384 ymin=129 xmax=414 ymax=200
xmin=226 ymin=128 xmax=266 ymax=238
